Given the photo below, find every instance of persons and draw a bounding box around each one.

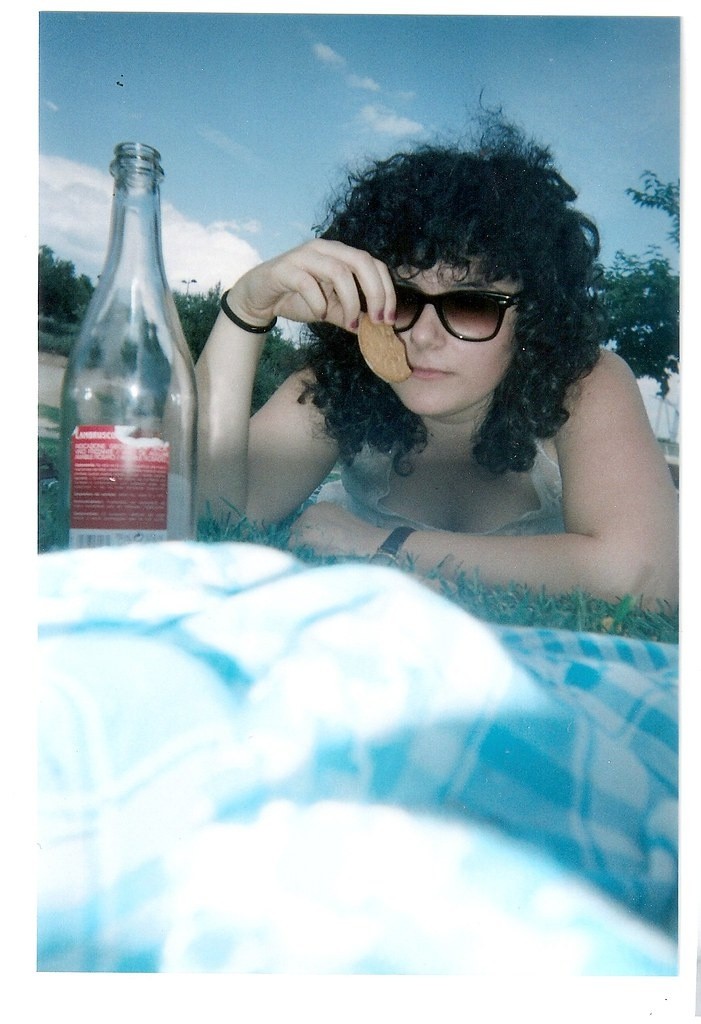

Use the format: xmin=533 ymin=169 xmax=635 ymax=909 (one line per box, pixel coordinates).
xmin=193 ymin=148 xmax=679 ymax=617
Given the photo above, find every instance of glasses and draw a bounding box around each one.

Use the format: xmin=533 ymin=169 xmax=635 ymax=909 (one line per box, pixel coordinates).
xmin=360 ymin=282 xmax=528 ymax=342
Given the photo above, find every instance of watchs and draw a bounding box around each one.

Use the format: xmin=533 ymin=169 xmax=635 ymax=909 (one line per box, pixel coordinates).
xmin=369 ymin=526 xmax=417 ymax=568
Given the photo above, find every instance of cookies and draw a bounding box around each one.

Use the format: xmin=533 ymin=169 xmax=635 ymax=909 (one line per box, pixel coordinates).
xmin=357 ymin=313 xmax=412 ymax=383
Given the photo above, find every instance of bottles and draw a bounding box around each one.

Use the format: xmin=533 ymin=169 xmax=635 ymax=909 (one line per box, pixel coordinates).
xmin=60 ymin=143 xmax=199 ymax=549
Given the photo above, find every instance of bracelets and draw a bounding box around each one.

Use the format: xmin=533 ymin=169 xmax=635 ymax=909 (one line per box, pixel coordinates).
xmin=220 ymin=289 xmax=278 ymax=334
